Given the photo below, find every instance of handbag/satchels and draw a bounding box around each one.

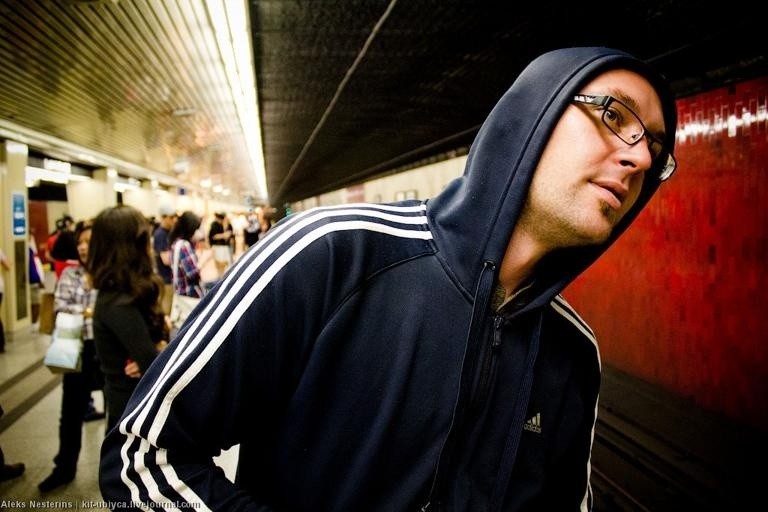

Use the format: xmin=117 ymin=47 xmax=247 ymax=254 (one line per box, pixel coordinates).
xmin=170 ymin=294 xmax=202 ymax=329
xmin=44 ymin=312 xmax=86 ymax=372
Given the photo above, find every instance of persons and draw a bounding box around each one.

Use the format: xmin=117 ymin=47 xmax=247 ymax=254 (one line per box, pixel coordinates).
xmin=96 ymin=45 xmax=677 ymax=510
xmin=0 ymin=449 xmax=26 ymax=483
xmin=39 ymin=204 xmax=292 ymax=302
xmin=0 ymin=247 xmax=10 ymax=357
xmin=86 ymin=204 xmax=171 ymax=430
xmin=37 ymin=224 xmax=96 ymax=494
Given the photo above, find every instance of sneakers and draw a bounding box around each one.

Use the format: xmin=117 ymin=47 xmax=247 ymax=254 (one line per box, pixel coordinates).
xmin=38 ymin=468 xmax=76 ymax=493
xmin=0 ymin=463 xmax=25 ymax=481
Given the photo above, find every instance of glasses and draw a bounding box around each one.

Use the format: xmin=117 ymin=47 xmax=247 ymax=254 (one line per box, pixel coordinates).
xmin=574 ymin=95 xmax=676 ymax=182
